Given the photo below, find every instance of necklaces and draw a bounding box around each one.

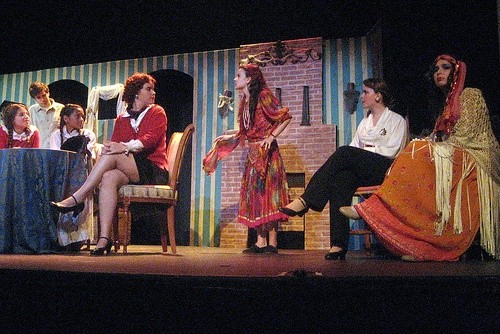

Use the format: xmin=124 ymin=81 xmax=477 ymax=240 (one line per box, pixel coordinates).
xmin=242 ymin=99 xmax=250 ymax=130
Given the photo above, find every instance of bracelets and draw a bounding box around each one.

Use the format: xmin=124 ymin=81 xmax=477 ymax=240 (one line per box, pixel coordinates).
xmin=270 ymin=133 xmax=276 ymax=138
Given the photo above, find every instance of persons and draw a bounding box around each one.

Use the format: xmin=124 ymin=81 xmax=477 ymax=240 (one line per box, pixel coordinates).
xmin=50 ymin=102 xmax=97 ymax=160
xmin=0 ymin=103 xmax=39 ymax=149
xmin=51 ymin=72 xmax=170 ymax=257
xmin=201 ymin=54 xmax=291 ymax=254
xmin=339 ymin=54 xmax=500 ymax=262
xmin=27 ymin=81 xmax=65 ymax=150
xmin=279 ymin=77 xmax=406 ymax=260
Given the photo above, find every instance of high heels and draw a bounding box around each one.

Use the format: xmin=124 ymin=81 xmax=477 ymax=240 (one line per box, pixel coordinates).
xmin=278 ymin=198 xmax=309 ymax=217
xmin=325 ymin=248 xmax=348 ymax=259
xmin=90 ymin=237 xmax=113 ymax=256
xmin=50 ymin=195 xmax=85 ymax=218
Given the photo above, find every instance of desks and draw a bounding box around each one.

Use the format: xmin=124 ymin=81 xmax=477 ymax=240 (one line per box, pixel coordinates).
xmin=0 ymin=148 xmax=99 ymax=253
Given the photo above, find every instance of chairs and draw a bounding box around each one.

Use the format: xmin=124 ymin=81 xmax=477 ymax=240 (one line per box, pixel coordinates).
xmin=349 ymin=115 xmax=411 ymax=248
xmin=110 ymin=122 xmax=194 ymax=255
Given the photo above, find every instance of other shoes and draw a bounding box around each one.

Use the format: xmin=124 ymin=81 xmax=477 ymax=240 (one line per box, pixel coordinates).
xmin=401 ymin=255 xmax=417 ymax=262
xmin=339 ymin=206 xmax=362 ymax=220
xmin=263 ymin=246 xmax=278 ymax=253
xmin=243 ymin=245 xmax=266 ymax=253
